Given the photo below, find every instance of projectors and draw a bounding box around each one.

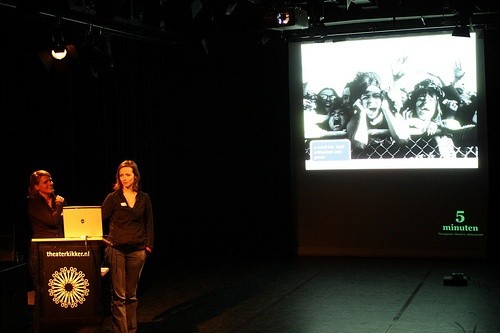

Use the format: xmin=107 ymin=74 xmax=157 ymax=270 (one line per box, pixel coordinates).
xmin=263 ymin=7 xmax=308 ymax=29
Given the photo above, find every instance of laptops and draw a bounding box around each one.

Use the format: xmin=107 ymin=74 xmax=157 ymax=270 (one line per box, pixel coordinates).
xmin=63 ymin=206 xmax=103 ymax=237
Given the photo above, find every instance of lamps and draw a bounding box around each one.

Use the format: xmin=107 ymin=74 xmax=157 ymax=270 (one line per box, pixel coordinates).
xmin=48 ymin=18 xmax=75 ymax=65
xmin=80 ymin=23 xmax=115 ymax=71
xmin=448 ymin=0 xmax=477 ymax=37
xmin=273 ymin=3 xmax=296 ymax=26
xmin=306 ymin=0 xmax=327 ymax=39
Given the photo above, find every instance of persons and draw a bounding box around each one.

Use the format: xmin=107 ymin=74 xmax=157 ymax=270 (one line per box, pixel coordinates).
xmin=27 ymin=170 xmax=67 ymax=333
xmin=101 ymin=159 xmax=154 ymax=333
xmin=302 ymin=56 xmax=477 ymax=150
